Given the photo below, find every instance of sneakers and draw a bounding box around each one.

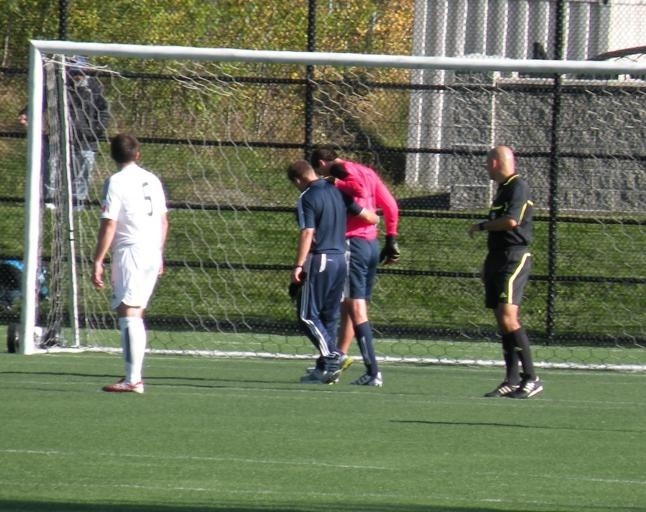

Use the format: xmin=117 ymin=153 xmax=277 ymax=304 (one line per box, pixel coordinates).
xmin=300 ymin=349 xmax=353 ymax=386
xmin=484 ymin=372 xmax=543 ymax=400
xmin=347 ymin=371 xmax=383 ymax=388
xmin=102 ymin=377 xmax=145 ymax=394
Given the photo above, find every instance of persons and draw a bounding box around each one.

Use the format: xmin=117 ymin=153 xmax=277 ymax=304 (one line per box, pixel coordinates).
xmin=301 ymin=146 xmax=401 ymax=386
xmin=89 ymin=133 xmax=169 ymax=395
xmin=467 ymin=145 xmax=545 ymax=398
xmin=282 ymin=159 xmax=381 ymax=383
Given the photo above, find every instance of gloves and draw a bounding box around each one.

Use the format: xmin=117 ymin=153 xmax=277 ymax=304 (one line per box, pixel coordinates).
xmin=378 ymin=235 xmax=401 ymax=267
xmin=288 ymin=271 xmax=308 ymax=304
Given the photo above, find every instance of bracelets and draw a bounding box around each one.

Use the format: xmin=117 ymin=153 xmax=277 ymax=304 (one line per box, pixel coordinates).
xmin=292 ymin=264 xmax=305 ymax=270
xmin=478 ymin=221 xmax=485 ymax=232
